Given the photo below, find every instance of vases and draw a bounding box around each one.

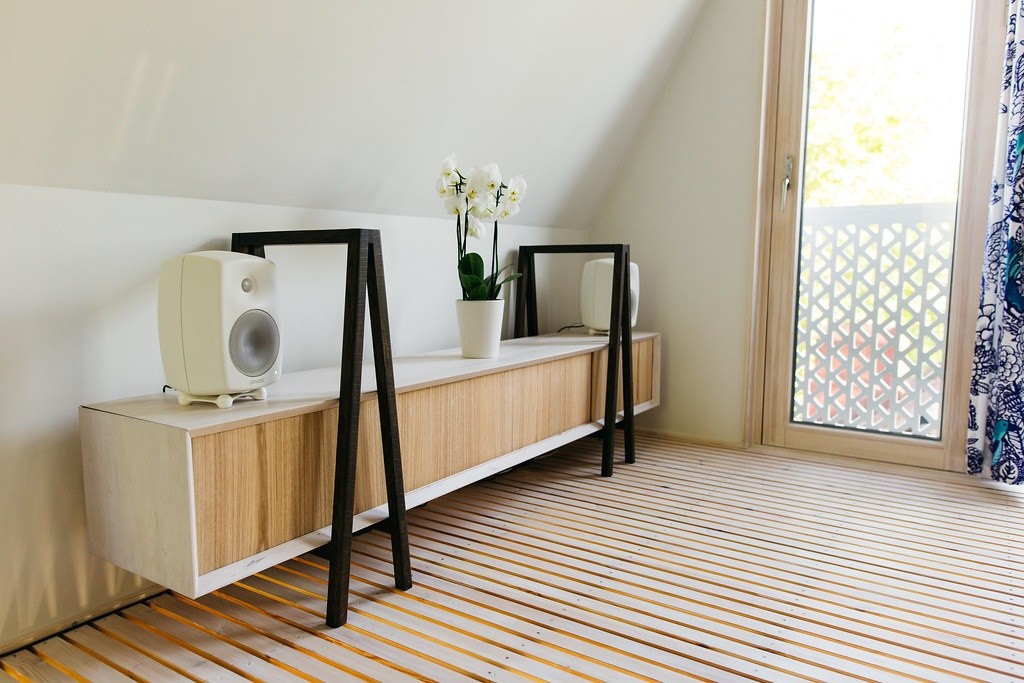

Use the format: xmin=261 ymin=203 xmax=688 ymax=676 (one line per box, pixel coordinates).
xmin=458 ymin=299 xmax=504 ymax=357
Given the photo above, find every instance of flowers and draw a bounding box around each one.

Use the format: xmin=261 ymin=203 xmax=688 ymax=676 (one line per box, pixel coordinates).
xmin=435 ymin=155 xmax=527 ymax=301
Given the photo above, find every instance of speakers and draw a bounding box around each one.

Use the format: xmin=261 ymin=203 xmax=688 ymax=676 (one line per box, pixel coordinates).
xmin=580 ymin=258 xmax=639 ymax=331
xmin=159 ymin=251 xmax=283 ymax=398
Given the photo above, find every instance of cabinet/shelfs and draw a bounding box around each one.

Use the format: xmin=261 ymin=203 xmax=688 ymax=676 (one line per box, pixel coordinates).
xmin=79 ymin=330 xmax=661 ymax=599
